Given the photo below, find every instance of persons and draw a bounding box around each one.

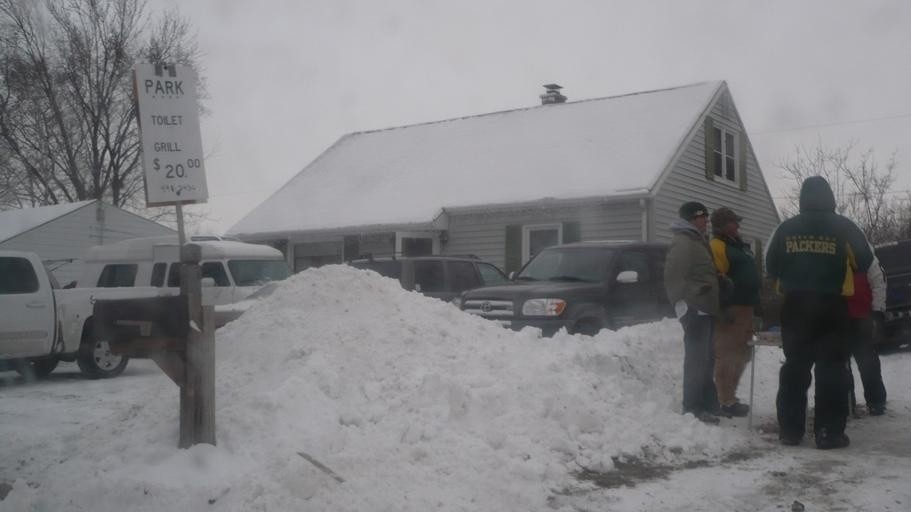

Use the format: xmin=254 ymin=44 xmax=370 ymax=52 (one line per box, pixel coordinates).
xmin=663 ymin=202 xmax=733 ymax=425
xmin=842 ymin=240 xmax=887 ymax=421
xmin=708 ymin=207 xmax=761 ymax=417
xmin=763 ymin=176 xmax=889 ymax=450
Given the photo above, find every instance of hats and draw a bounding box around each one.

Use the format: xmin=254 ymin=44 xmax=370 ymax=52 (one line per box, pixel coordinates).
xmin=712 ymin=207 xmax=742 ymax=222
xmin=679 ymin=201 xmax=710 ymax=220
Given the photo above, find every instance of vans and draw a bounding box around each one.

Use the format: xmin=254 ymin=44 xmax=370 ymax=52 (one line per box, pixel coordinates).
xmin=340 ymin=255 xmax=510 ymax=303
xmin=77 ymin=237 xmax=292 ymax=302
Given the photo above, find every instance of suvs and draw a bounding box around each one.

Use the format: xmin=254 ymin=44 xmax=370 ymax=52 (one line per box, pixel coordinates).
xmin=459 ymin=239 xmax=672 ymax=336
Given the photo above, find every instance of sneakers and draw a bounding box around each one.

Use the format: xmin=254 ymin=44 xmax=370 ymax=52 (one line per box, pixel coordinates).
xmin=703 ymin=399 xmax=733 ymax=418
xmin=868 ymin=403 xmax=883 ymax=415
xmin=722 ymin=402 xmax=749 ymax=417
xmin=816 ymin=428 xmax=850 ymax=450
xmin=778 ymin=431 xmax=802 ymax=446
xmin=697 ymin=410 xmax=720 ymax=426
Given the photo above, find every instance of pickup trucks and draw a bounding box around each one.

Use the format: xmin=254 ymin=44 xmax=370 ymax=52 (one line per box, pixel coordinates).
xmin=0 ymin=248 xmax=182 ymax=378
